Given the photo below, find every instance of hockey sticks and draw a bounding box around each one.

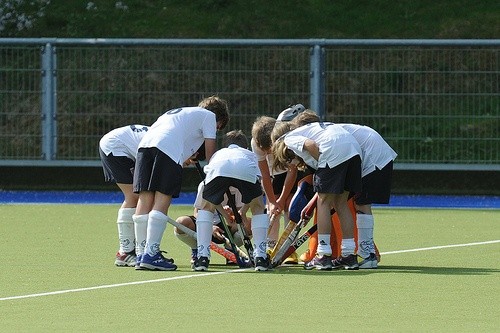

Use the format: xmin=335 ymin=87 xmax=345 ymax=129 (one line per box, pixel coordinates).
xmin=167 ymin=153 xmax=356 ymax=268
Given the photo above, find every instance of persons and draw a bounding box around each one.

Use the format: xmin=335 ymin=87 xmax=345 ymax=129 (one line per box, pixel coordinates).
xmin=99 ymin=124 xmax=206 ymax=267
xmin=270 ymin=121 xmax=362 ymax=271
xmin=192 ymin=129 xmax=272 ymax=272
xmin=270 ymin=104 xmax=381 ymax=267
xmin=131 ymin=95 xmax=231 ymax=270
xmin=289 ymin=109 xmax=399 ymax=269
xmin=175 ymin=205 xmax=255 ymax=266
xmin=251 ymin=115 xmax=303 ymax=267
